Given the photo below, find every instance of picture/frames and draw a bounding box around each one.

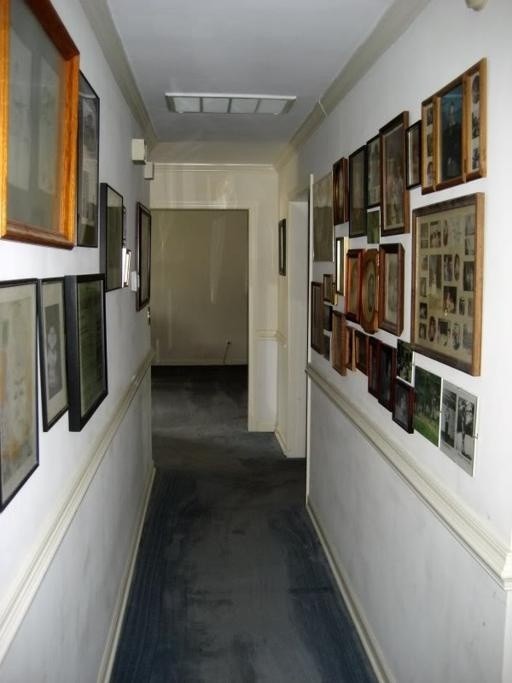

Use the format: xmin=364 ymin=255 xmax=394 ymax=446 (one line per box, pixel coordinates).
xmin=279 ymin=218 xmax=287 ymax=276
